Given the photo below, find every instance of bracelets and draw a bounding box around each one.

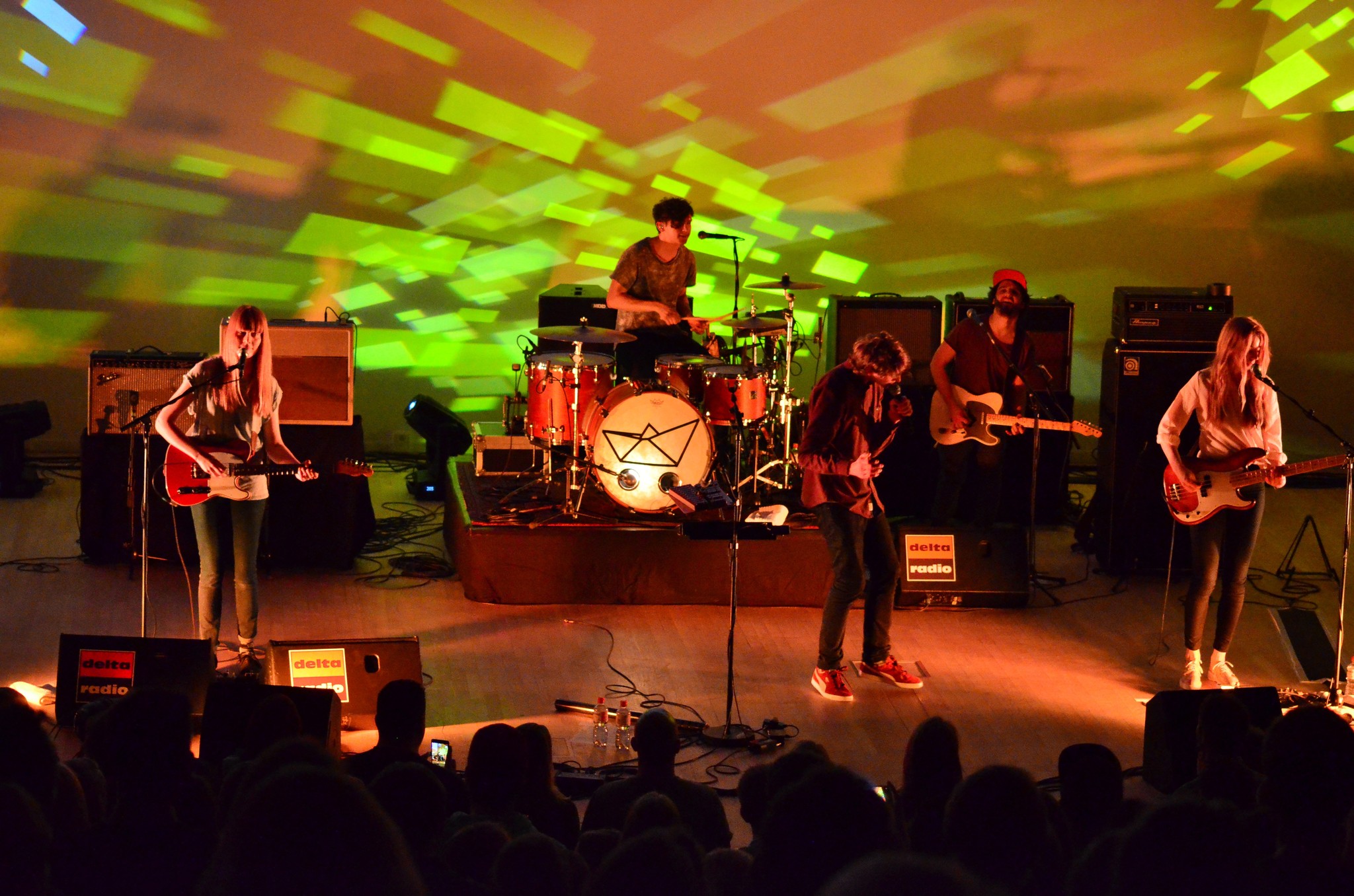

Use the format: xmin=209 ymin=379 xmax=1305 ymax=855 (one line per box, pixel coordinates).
xmin=195 ymin=452 xmax=198 ymax=461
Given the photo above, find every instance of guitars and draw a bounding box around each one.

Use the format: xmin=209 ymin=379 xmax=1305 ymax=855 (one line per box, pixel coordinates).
xmin=164 ymin=436 xmax=374 ymax=508
xmin=1161 ymin=446 xmax=1354 ymax=525
xmin=926 ymin=385 xmax=1104 ymax=447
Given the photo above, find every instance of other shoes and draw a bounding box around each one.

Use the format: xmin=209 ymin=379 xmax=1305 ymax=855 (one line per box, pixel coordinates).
xmin=1004 ymin=534 xmax=1013 ymax=548
xmin=240 ymin=652 xmax=262 ymax=671
xmin=214 ymin=655 xmax=218 ymax=668
xmin=979 ymin=540 xmax=991 ymax=557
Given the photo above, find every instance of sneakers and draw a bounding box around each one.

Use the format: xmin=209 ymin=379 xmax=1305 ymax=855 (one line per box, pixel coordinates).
xmin=1207 ymin=660 xmax=1242 ymax=687
xmin=1180 ymin=661 xmax=1203 ymax=690
xmin=810 ymin=666 xmax=853 ymax=701
xmin=860 ymin=654 xmax=923 ymax=689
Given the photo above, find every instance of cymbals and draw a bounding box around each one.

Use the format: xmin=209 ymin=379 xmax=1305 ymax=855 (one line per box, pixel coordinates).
xmin=719 ymin=313 xmax=787 ymax=329
xmin=529 ymin=325 xmax=638 ymax=344
xmin=742 ymin=282 xmax=828 ymax=290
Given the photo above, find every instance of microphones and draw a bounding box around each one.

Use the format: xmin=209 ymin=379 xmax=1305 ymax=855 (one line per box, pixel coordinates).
xmin=728 ymin=343 xmax=761 ymax=353
xmin=623 ymin=472 xmax=637 ymax=487
xmin=1251 ymin=363 xmax=1263 ymax=380
xmin=698 ymin=231 xmax=739 ymax=239
xmin=238 ymin=347 xmax=248 ymax=376
xmin=527 ymin=338 xmax=537 ymax=349
xmin=966 ymin=308 xmax=988 ymax=332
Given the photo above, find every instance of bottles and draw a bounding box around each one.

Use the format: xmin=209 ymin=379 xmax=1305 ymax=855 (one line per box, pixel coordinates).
xmin=592 ymin=698 xmax=608 ymax=746
xmin=615 ymin=700 xmax=631 ymax=749
xmin=790 ymin=443 xmax=802 ymax=478
xmin=1345 ymin=656 xmax=1354 ymax=695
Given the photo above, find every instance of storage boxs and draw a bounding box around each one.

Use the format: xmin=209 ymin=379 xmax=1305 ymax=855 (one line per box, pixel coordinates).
xmin=471 ymin=421 xmax=544 ymax=476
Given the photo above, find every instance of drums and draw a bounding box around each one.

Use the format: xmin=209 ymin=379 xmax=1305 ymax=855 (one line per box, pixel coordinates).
xmin=653 ymin=351 xmax=727 ymax=407
xmin=525 ymin=350 xmax=618 ymax=446
xmin=583 ymin=378 xmax=716 ymax=513
xmin=698 ymin=361 xmax=773 ymax=428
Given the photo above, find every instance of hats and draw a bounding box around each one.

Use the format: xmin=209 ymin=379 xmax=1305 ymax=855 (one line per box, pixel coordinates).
xmin=993 ymin=269 xmax=1028 ymax=289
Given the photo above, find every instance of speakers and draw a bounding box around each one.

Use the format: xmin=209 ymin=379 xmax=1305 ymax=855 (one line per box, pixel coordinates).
xmin=945 ymin=293 xmax=1073 ymax=397
xmin=897 ymin=527 xmax=1028 ymax=608
xmin=264 ymin=635 xmax=423 ymax=730
xmin=219 ymin=317 xmax=355 ymax=426
xmin=1142 ymin=686 xmax=1282 ymax=796
xmin=825 ymin=295 xmax=942 ymax=396
xmin=87 ymin=351 xmax=209 ymax=435
xmin=1093 ymin=338 xmax=1224 ymax=575
xmin=55 ymin=634 xmax=215 ymax=736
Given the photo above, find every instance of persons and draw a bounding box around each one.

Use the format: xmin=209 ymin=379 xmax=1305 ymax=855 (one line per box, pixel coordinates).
xmin=1156 ymin=316 xmax=1288 ymax=690
xmin=606 ymin=199 xmax=710 ymax=380
xmin=798 ymin=331 xmax=924 ymax=702
xmin=155 ymin=305 xmax=320 ymax=675
xmin=0 ymin=679 xmax=1354 ymax=896
xmin=930 ymin=270 xmax=1036 ymax=525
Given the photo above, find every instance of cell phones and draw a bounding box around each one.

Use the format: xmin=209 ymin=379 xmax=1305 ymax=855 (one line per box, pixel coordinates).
xmin=430 ymin=739 xmax=449 ymax=768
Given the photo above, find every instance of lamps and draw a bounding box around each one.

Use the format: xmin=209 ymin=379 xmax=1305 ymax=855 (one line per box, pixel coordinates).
xmin=0 ymin=398 xmax=54 ymax=499
xmin=402 ymin=393 xmax=473 ymax=502
